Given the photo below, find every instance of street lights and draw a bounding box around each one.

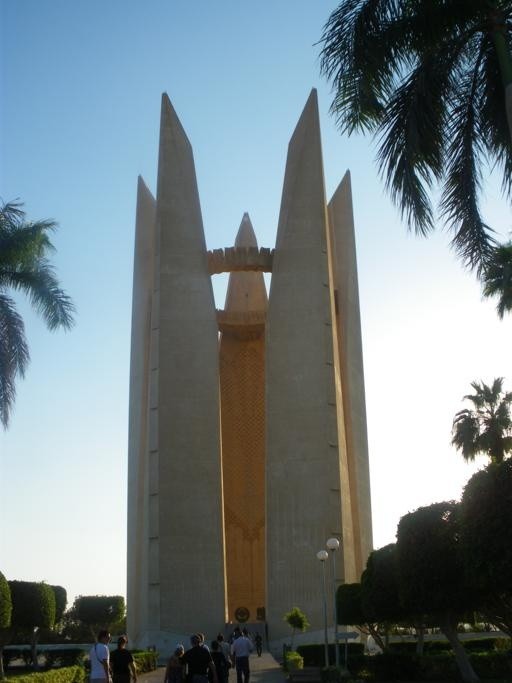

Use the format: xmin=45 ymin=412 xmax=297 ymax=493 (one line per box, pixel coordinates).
xmin=326 ymin=537 xmax=341 ymax=667
xmin=316 ymin=549 xmax=329 ymax=666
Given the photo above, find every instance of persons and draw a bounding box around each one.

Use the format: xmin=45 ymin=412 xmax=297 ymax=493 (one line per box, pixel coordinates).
xmin=254 ymin=632 xmax=264 ymax=657
xmin=88 ymin=630 xmax=111 ymax=683
xmin=160 ymin=621 xmax=254 ymax=682
xmin=108 ymin=636 xmax=136 ymax=683
